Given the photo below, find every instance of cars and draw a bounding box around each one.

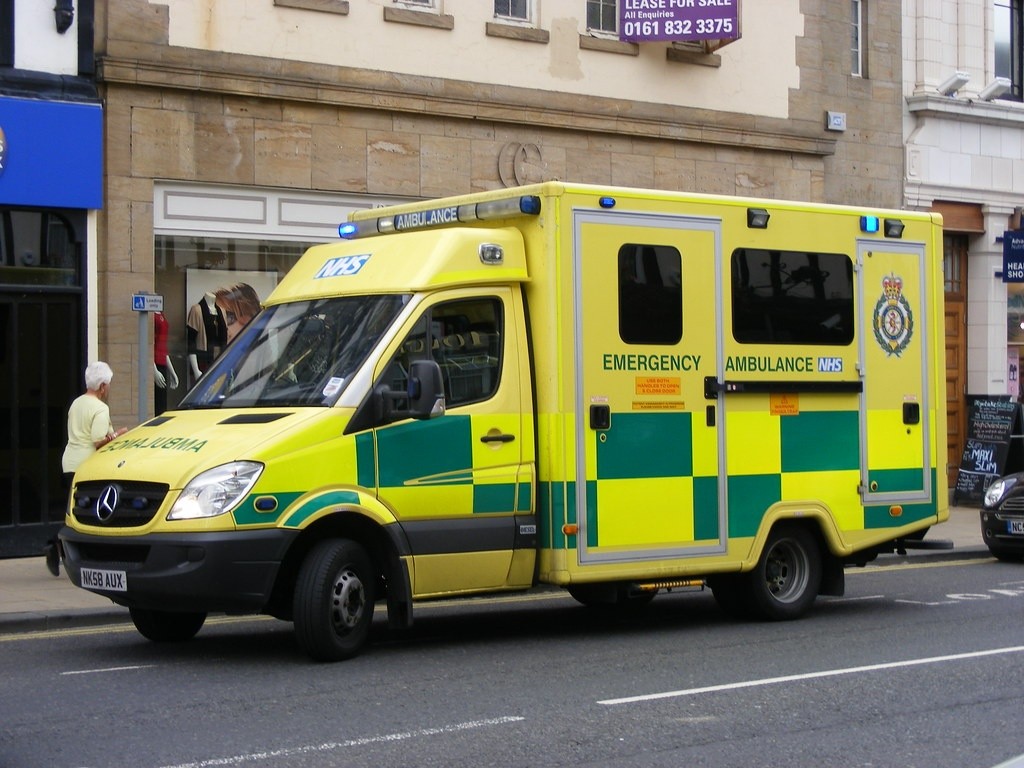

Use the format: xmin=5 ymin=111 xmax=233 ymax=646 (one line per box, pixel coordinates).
xmin=980 ymin=471 xmax=1024 ymax=567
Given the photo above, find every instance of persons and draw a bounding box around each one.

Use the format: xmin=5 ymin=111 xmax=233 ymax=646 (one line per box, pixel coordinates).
xmin=286 ymin=313 xmax=332 ymax=387
xmin=211 ymin=281 xmax=260 ymax=340
xmin=46 ymin=361 xmax=128 ymax=576
xmin=153 ymin=305 xmax=179 ymax=417
xmin=186 ymin=292 xmax=227 ymax=384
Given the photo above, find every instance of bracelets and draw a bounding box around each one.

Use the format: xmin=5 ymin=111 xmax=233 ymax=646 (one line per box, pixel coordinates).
xmin=106 ymin=432 xmax=117 ymax=443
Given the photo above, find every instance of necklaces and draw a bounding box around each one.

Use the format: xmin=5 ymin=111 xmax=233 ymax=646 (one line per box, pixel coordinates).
xmin=207 ymin=308 xmax=218 ymax=326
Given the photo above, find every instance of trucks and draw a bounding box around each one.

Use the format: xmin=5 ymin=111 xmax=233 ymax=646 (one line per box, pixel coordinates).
xmin=52 ymin=180 xmax=948 ymax=663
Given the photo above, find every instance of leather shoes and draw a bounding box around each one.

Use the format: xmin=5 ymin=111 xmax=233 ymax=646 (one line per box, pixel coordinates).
xmin=43 ymin=542 xmax=61 ymax=580
